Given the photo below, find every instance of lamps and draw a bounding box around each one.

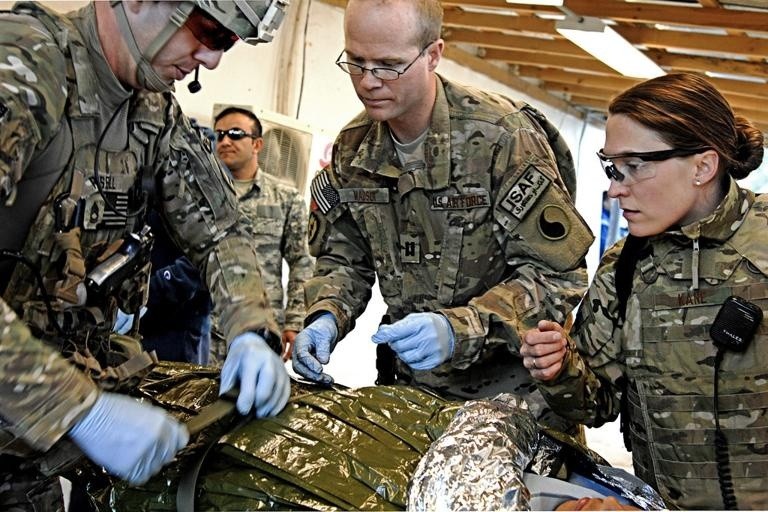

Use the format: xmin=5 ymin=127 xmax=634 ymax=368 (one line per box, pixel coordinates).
xmin=553 ymin=14 xmax=674 ymax=83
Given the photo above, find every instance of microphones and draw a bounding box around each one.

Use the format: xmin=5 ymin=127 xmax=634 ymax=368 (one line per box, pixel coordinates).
xmin=188 ymin=65 xmax=201 ymax=93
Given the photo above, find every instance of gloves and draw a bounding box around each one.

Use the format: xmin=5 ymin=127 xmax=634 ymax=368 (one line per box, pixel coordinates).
xmin=372 ymin=311 xmax=454 ymax=371
xmin=217 ymin=331 xmax=291 ymax=418
xmin=291 ymin=313 xmax=346 ymax=385
xmin=69 ymin=392 xmax=190 ymax=486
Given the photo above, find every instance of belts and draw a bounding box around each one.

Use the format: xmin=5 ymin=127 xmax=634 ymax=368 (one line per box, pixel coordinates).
xmin=176 ymin=388 xmax=241 ymax=512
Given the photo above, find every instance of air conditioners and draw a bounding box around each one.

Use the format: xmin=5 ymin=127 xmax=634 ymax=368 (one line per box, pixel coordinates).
xmin=208 ymin=99 xmax=348 ymax=215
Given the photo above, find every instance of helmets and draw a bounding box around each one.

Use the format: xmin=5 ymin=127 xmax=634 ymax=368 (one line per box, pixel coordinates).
xmin=195 ymin=1 xmax=286 ymax=46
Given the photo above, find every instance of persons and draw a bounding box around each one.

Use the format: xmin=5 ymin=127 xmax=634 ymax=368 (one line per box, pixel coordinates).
xmin=0 ymin=1 xmax=294 ymax=511
xmin=282 ymin=2 xmax=589 ymax=405
xmin=202 ymin=104 xmax=311 ymax=360
xmin=518 ymin=73 xmax=767 ymax=510
xmin=71 ymin=358 xmax=673 ymax=509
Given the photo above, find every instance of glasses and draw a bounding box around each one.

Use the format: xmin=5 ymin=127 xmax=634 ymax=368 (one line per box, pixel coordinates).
xmin=214 ymin=128 xmax=254 ymax=142
xmin=336 ymin=43 xmax=431 ymax=81
xmin=595 ymin=147 xmax=684 ymax=186
xmin=183 ymin=4 xmax=238 ymax=52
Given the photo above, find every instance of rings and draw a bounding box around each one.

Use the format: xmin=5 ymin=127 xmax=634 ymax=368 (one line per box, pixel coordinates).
xmin=529 ymin=355 xmax=544 ymax=370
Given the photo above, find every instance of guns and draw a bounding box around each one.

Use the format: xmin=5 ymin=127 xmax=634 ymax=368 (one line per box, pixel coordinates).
xmin=86 ymin=225 xmax=155 ymax=340
